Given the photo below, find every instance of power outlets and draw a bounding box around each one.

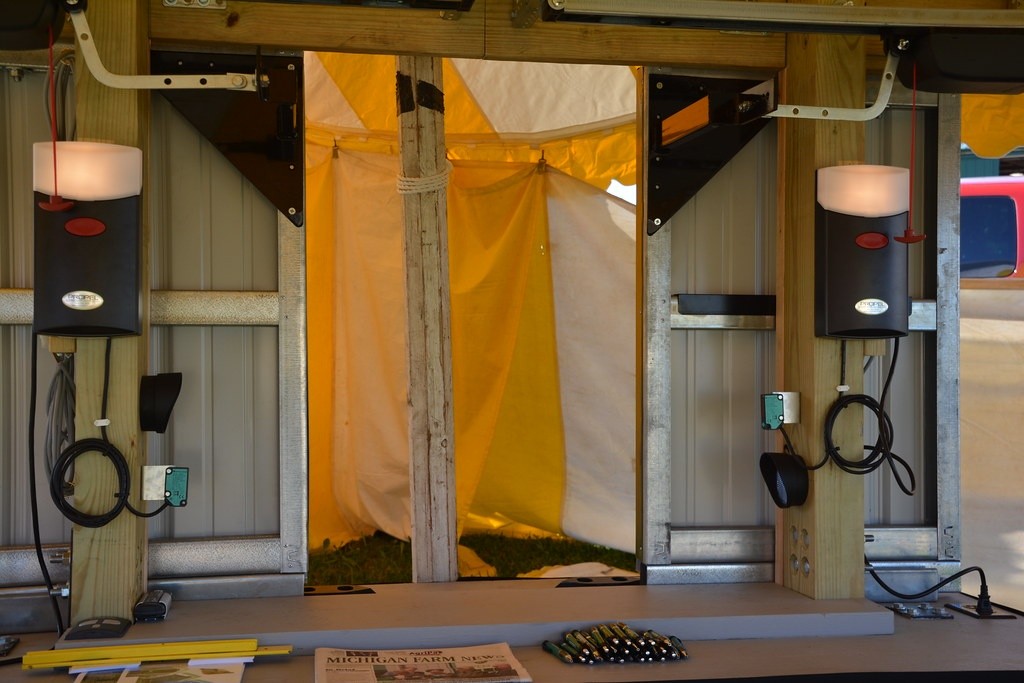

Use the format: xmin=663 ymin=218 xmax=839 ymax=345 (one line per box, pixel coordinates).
xmin=944 ymin=599 xmax=1017 ymax=619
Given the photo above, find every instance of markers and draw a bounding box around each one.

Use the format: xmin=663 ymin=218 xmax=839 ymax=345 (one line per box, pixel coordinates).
xmin=542 ymin=623 xmax=688 ymax=666
